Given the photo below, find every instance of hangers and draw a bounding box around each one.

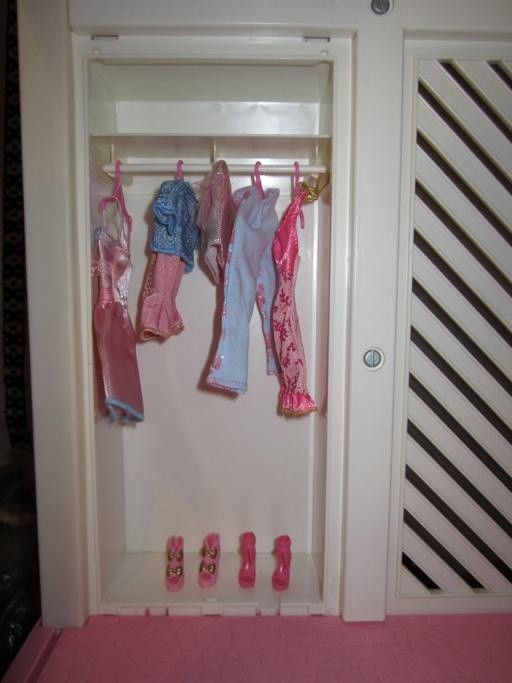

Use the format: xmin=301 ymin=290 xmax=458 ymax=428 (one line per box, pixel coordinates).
xmin=99 ymin=161 xmax=304 ymax=229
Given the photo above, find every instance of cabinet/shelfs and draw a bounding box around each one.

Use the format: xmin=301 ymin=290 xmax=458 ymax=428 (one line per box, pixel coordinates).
xmin=69 ymin=1 xmax=357 ymax=618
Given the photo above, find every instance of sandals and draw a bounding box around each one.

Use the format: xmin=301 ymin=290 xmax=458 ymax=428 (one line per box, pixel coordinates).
xmin=165 ymin=536 xmax=184 ymax=590
xmin=197 ymin=534 xmax=219 ymax=588
xmin=273 ymin=536 xmax=290 ymax=591
xmin=240 ymin=533 xmax=256 ymax=588
